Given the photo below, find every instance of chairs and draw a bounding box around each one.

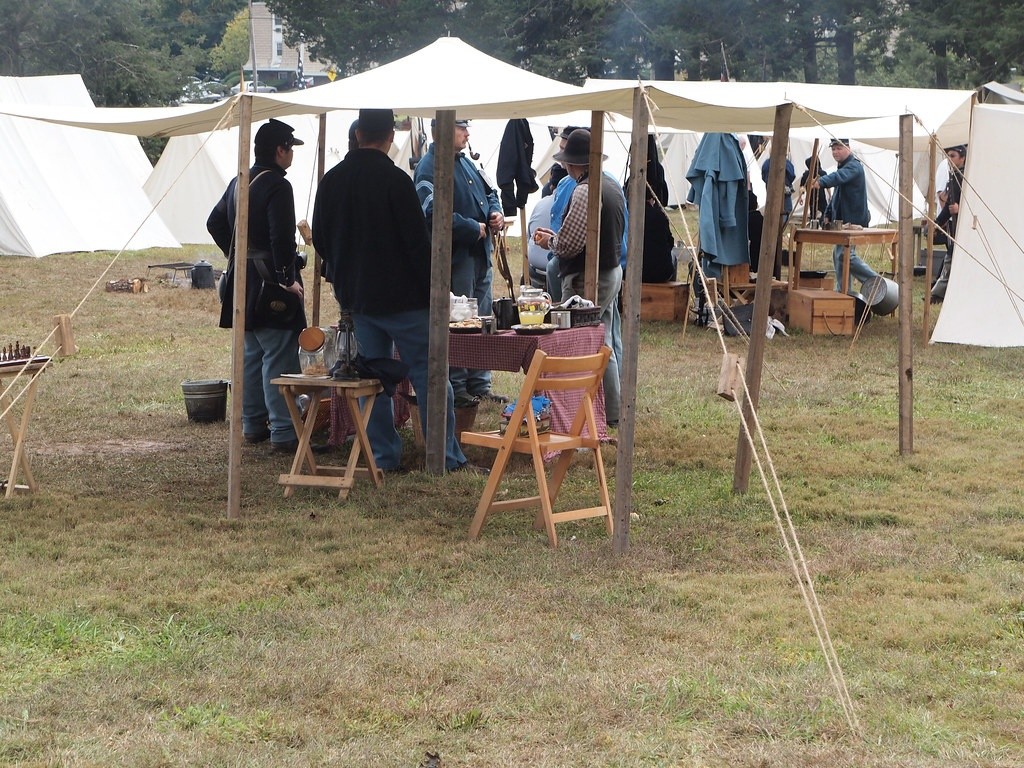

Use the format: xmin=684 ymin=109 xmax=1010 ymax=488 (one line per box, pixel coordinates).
xmin=460 ymin=346 xmax=613 ymax=545
xmin=700 ymin=257 xmax=783 ymax=309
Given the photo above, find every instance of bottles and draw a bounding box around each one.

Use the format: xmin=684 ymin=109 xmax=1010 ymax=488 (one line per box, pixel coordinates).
xmin=298 ymin=319 xmax=357 ymax=377
xmin=450 ymin=297 xmax=478 ymax=322
xmin=822 ymin=206 xmax=831 ymax=230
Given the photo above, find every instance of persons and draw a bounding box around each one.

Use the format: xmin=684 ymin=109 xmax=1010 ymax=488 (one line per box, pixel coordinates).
xmin=312 ymin=109 xmax=490 ymax=474
xmin=761 ymin=147 xmax=795 ymax=223
xmin=934 ymin=145 xmax=968 ymax=277
xmin=812 ymin=139 xmax=880 ymax=292
xmin=207 ymin=116 xmax=307 ymax=452
xmin=642 ymin=181 xmax=678 ymax=282
xmin=526 ymin=126 xmax=629 ymax=429
xmin=413 ymin=118 xmax=510 ymax=405
xmin=798 ymin=157 xmax=831 ymax=220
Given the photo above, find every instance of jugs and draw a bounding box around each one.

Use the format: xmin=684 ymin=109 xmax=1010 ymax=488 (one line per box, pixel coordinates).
xmin=518 ymin=288 xmax=552 ymax=324
xmin=492 ymin=297 xmax=515 ymax=329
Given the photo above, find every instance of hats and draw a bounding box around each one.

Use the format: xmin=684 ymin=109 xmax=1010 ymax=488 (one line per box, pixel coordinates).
xmin=431 ymin=119 xmax=470 ymax=128
xmin=554 ymin=125 xmax=591 ymax=140
xmin=944 ymin=145 xmax=966 ymax=157
xmin=829 ymin=139 xmax=849 ymax=147
xmin=254 ymin=119 xmax=304 ymax=146
xmin=554 ymin=130 xmax=608 ymax=165
xmin=359 ymin=109 xmax=396 ymax=131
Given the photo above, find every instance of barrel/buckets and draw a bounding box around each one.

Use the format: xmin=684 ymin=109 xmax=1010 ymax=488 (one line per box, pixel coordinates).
xmin=922 ymin=248 xmax=945 ymax=276
xmin=860 ymin=276 xmax=900 ymax=316
xmin=180 ymin=379 xmax=228 ymax=424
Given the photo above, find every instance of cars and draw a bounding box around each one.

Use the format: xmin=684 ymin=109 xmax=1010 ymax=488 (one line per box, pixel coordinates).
xmin=198 ymin=81 xmax=227 ymax=98
xmin=179 ymin=88 xmax=223 ymax=104
xmin=178 ymin=76 xmax=202 ymax=85
xmin=230 ymin=81 xmax=277 ymax=94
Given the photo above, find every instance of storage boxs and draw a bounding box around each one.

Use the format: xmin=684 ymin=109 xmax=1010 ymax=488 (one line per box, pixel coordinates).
xmin=788 ymin=289 xmax=856 ymax=336
xmin=543 ymin=306 xmax=601 ymax=328
xmin=639 ymin=281 xmax=690 ymax=322
xmin=791 ymin=277 xmax=834 ymax=291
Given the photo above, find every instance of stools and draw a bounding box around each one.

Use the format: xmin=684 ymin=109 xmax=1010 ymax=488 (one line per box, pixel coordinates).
xmin=270 ymin=376 xmax=386 ymax=503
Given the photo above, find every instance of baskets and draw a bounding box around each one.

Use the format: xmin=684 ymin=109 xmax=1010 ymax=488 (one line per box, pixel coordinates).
xmin=544 ymin=304 xmax=602 ymax=327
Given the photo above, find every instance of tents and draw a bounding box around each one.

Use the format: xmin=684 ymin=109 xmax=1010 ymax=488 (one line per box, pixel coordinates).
xmin=0 ymin=37 xmax=1023 ymax=349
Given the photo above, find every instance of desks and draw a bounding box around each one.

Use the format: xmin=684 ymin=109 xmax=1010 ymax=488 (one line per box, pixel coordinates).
xmin=788 ymin=225 xmax=900 ymax=322
xmin=0 ymin=354 xmax=53 ymax=501
xmin=326 ymin=321 xmax=605 ymax=466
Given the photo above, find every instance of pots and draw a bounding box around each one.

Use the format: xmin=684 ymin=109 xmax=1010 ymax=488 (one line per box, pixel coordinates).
xmin=846 ymin=290 xmax=871 ymax=327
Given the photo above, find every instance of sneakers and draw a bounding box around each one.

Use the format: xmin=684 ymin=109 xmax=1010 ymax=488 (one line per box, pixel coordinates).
xmin=244 ymin=421 xmax=272 ymax=443
xmin=271 ymin=435 xmax=329 ymax=456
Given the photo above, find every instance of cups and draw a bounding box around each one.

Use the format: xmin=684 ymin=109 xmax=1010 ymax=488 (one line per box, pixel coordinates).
xmin=810 ymin=220 xmax=819 ymax=229
xmin=551 ymin=311 xmax=571 ymax=329
xmin=834 ymin=219 xmax=843 ymax=230
xmin=481 ymin=318 xmax=497 ymax=334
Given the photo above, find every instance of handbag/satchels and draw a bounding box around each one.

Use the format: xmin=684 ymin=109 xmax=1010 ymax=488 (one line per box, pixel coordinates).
xmin=255 ymin=280 xmax=298 ymax=324
xmin=765 ymin=316 xmax=789 ymax=341
xmin=721 ymin=303 xmax=753 ymax=336
xmin=784 ymin=183 xmax=796 ymax=195
xmin=217 ymin=269 xmax=227 ymax=302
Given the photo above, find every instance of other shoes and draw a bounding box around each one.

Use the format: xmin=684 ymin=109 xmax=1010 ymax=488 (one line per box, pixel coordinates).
xmin=479 ymin=390 xmax=509 ymax=405
xmin=400 ymin=447 xmax=490 ymax=475
xmin=921 ymin=295 xmax=943 ymax=304
xmin=607 ymin=419 xmax=618 ymax=428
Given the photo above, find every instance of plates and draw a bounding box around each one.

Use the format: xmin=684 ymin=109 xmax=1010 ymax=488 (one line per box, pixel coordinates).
xmin=509 ymin=323 xmax=559 ymax=334
xmin=448 ymin=327 xmax=482 ymax=334
